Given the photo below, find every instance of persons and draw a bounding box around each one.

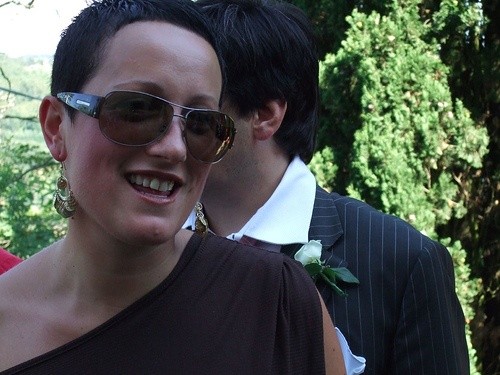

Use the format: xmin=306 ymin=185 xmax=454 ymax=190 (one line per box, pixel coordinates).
xmin=0 ymin=0 xmax=346 ymax=375
xmin=181 ymin=0 xmax=470 ymax=375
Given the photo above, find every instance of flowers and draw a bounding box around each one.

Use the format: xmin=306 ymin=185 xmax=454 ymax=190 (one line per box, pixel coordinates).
xmin=294 ymin=239 xmax=359 ymax=296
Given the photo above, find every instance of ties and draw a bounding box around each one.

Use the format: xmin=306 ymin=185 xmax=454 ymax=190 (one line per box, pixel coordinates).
xmin=239 ymin=235 xmax=258 ymax=246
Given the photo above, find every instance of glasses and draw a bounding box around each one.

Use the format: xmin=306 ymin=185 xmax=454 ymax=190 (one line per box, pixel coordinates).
xmin=57 ymin=90 xmax=237 ymax=164
